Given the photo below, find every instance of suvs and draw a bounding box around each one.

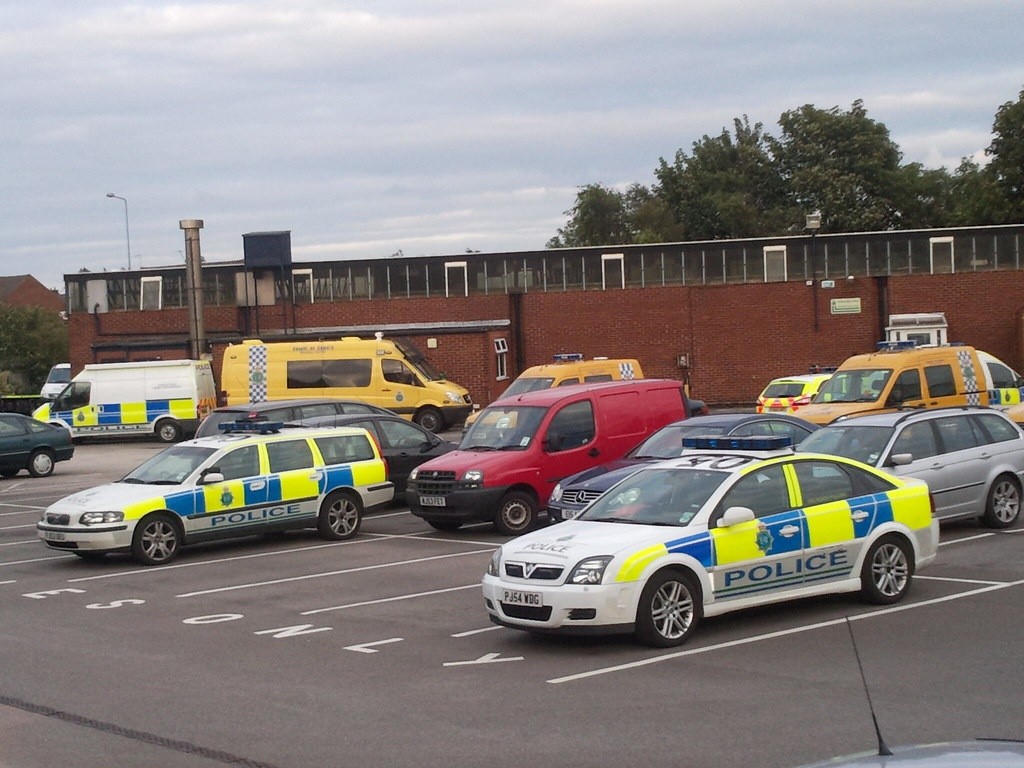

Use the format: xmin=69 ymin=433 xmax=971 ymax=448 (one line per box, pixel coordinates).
xmin=404 ymin=377 xmax=690 ymax=536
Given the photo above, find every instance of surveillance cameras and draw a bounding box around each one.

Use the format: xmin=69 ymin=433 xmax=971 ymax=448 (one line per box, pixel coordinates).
xmin=59 ymin=311 xmax=66 ymax=316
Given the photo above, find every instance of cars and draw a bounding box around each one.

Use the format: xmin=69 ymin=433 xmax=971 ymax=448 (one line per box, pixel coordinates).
xmin=686 ymin=398 xmax=710 ymax=421
xmin=272 ymin=412 xmax=460 ymax=510
xmin=789 ymin=403 xmax=1023 ymax=531
xmin=0 ymin=412 xmax=75 ymax=478
xmin=755 ymin=365 xmax=865 ymax=416
xmin=36 ymin=414 xmax=397 ymax=568
xmin=191 ymin=400 xmax=441 ymax=468
xmin=546 ymin=413 xmax=870 ymax=522
xmin=860 ymin=348 xmax=1022 ymax=403
xmin=482 ymin=433 xmax=941 ymax=648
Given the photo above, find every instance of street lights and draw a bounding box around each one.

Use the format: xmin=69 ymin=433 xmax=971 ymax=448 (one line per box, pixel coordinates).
xmin=107 ymin=192 xmax=132 ymax=271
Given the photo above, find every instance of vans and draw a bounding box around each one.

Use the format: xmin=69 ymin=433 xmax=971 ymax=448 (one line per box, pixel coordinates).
xmin=459 ymin=353 xmax=645 ymax=446
xmin=33 ymin=358 xmax=218 ymax=442
xmin=39 ymin=362 xmax=71 ymax=401
xmin=220 ymin=331 xmax=473 ymax=437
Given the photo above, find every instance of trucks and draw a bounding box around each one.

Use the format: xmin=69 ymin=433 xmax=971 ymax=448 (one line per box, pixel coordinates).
xmin=791 ymin=340 xmax=1024 ymax=445
